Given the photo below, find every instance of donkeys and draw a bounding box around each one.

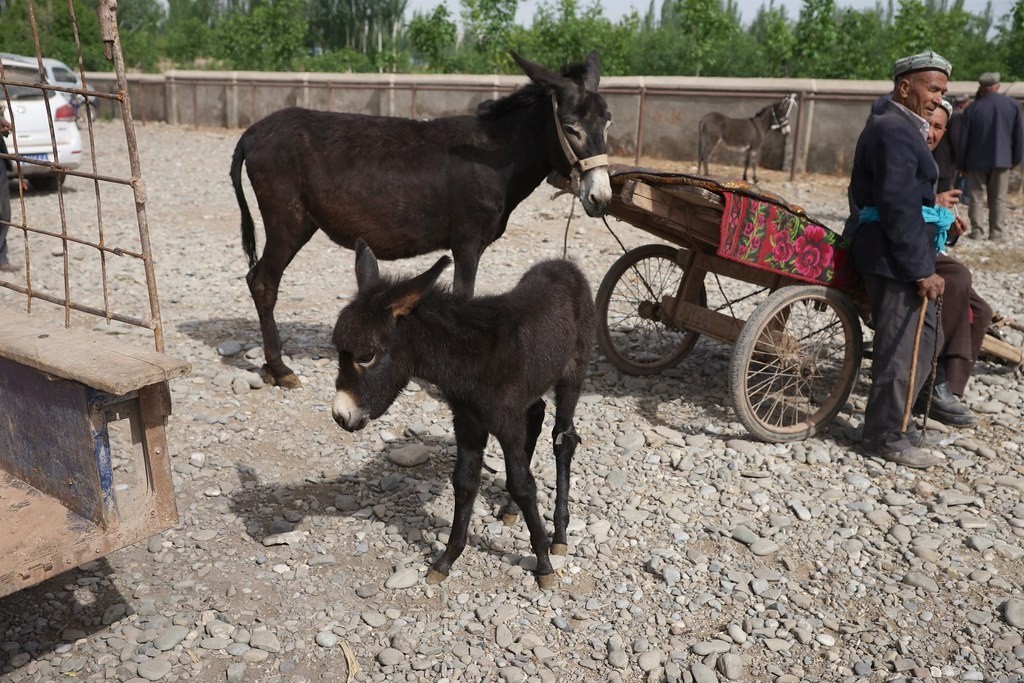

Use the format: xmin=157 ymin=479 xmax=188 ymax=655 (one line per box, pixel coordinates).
xmin=698 ymin=99 xmax=792 ymax=185
xmin=228 ymin=48 xmax=617 ymax=389
xmin=331 ymin=237 xmax=598 ymax=590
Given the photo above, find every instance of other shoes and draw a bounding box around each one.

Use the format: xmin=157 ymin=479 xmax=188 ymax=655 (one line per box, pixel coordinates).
xmin=909 ymin=429 xmax=941 ymax=446
xmin=882 ymin=446 xmax=939 ymax=468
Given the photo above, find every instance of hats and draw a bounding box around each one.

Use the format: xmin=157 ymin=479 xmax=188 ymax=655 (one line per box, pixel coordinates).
xmin=978 ymin=72 xmax=1001 ymax=86
xmin=894 ymin=50 xmax=952 ymax=81
xmin=955 ymin=93 xmax=969 ymax=101
xmin=938 ymin=96 xmax=954 ymax=119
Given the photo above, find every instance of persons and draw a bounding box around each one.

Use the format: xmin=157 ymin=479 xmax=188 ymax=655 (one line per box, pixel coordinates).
xmin=926 ymin=100 xmax=993 ymax=428
xmin=963 ymin=71 xmax=1024 ymax=240
xmin=0 ymin=105 xmax=20 ymax=272
xmin=931 ymin=93 xmax=971 ymax=195
xmin=844 ymin=49 xmax=951 ymax=470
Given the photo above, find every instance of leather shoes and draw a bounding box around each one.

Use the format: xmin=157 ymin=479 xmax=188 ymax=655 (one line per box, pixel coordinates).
xmin=916 ymin=382 xmax=976 ymax=427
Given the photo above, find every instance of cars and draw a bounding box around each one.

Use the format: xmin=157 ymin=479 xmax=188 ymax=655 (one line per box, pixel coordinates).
xmin=0 ymin=52 xmax=83 ymax=192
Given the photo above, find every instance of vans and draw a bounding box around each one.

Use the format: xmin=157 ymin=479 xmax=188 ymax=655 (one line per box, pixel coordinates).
xmin=28 ymin=57 xmax=101 ymax=129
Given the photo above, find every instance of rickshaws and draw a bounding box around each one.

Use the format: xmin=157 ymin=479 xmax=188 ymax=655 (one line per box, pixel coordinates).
xmin=543 ymin=161 xmax=1021 ymax=443
